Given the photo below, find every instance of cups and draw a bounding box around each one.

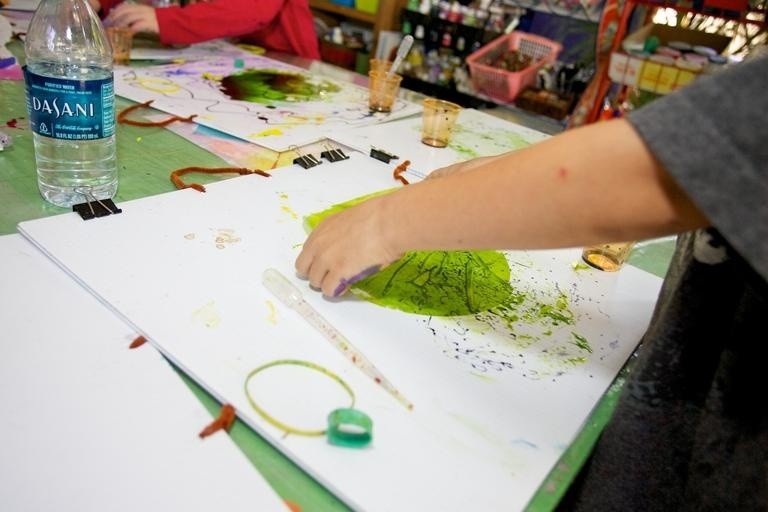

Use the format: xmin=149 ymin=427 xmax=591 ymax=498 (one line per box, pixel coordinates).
xmin=368 ymin=59 xmax=403 ymax=114
xmin=582 ymin=240 xmax=637 ymax=273
xmin=106 ymin=28 xmax=134 ymax=67
xmin=420 ymin=98 xmax=461 ymax=149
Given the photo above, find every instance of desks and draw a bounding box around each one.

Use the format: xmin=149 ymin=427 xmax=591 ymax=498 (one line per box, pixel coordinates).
xmin=0 ymin=10 xmax=695 ymax=512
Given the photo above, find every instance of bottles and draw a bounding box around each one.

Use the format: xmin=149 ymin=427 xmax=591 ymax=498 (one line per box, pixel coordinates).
xmin=21 ymin=0 xmax=119 ymax=210
xmin=397 ymin=0 xmax=527 ymax=93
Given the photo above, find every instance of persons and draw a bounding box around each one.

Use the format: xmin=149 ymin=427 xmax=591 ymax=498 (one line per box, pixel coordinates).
xmin=295 ymin=47 xmax=766 ymax=304
xmin=89 ymin=2 xmax=322 ymax=61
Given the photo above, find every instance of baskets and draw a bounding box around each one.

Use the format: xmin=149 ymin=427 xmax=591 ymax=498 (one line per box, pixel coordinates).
xmin=466 ymin=31 xmax=563 ymax=107
